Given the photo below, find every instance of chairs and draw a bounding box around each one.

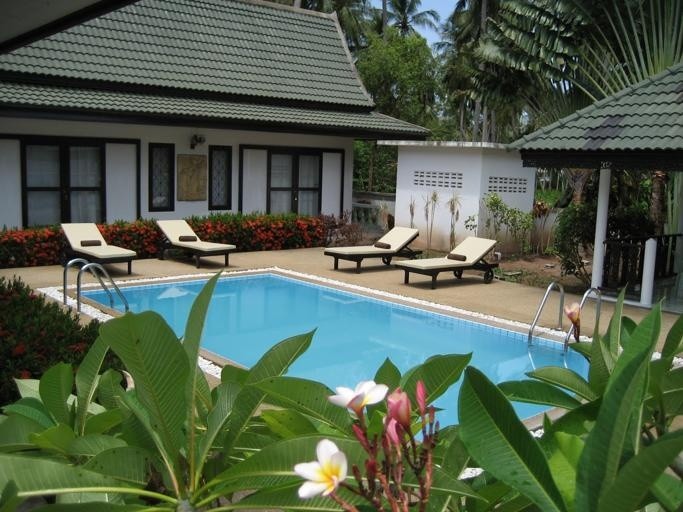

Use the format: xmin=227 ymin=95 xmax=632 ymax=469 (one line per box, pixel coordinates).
xmin=156 ymin=219 xmax=236 ymax=271
xmin=324 ymin=226 xmax=499 ymax=290
xmin=57 ymin=222 xmax=136 ymax=277
